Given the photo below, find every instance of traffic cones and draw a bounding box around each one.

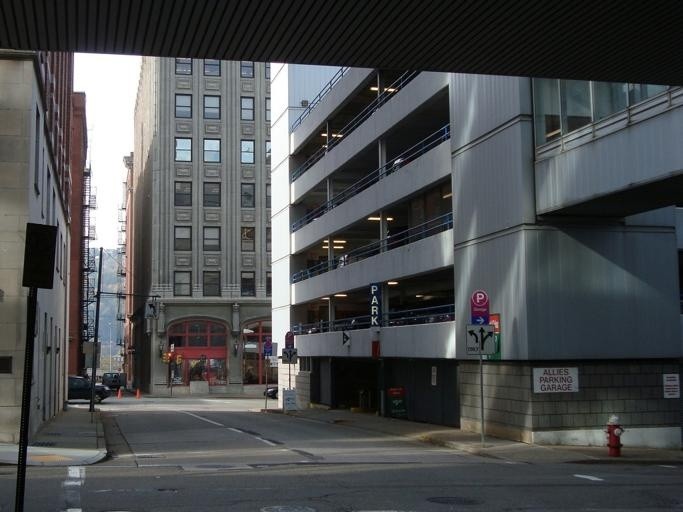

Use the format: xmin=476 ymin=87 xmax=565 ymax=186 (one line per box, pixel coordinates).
xmin=117 ymin=388 xmax=122 ymax=399
xmin=135 ymin=387 xmax=141 ymax=398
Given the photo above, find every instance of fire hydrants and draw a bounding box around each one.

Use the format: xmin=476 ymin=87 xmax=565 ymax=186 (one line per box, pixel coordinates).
xmin=605 ymin=415 xmax=624 ymax=456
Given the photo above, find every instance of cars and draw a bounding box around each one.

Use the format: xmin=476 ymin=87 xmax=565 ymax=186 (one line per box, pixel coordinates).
xmin=264 ymin=387 xmax=278 ymax=398
xmin=102 ymin=372 xmax=127 ymax=390
xmin=68 ymin=375 xmax=111 ymax=404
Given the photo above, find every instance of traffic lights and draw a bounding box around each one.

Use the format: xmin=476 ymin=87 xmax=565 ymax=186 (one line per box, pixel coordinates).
xmin=161 ymin=352 xmax=173 ymax=363
